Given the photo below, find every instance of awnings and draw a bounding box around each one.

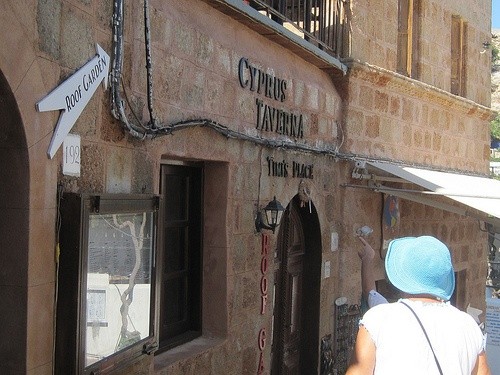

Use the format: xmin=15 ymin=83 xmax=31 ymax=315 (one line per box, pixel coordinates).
xmin=363 ymin=159 xmax=500 ymax=229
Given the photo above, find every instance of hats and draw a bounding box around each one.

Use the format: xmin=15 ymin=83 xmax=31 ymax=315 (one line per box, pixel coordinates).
xmin=384 ymin=234 xmax=455 ymax=300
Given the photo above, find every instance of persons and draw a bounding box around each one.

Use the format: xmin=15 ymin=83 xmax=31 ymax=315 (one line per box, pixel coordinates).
xmin=345 ymin=235 xmax=491 ymax=375
xmin=355 ymin=237 xmax=389 ymax=309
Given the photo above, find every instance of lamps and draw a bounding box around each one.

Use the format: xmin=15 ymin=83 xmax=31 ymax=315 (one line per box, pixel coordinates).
xmin=254 ymin=194 xmax=288 ymax=234
